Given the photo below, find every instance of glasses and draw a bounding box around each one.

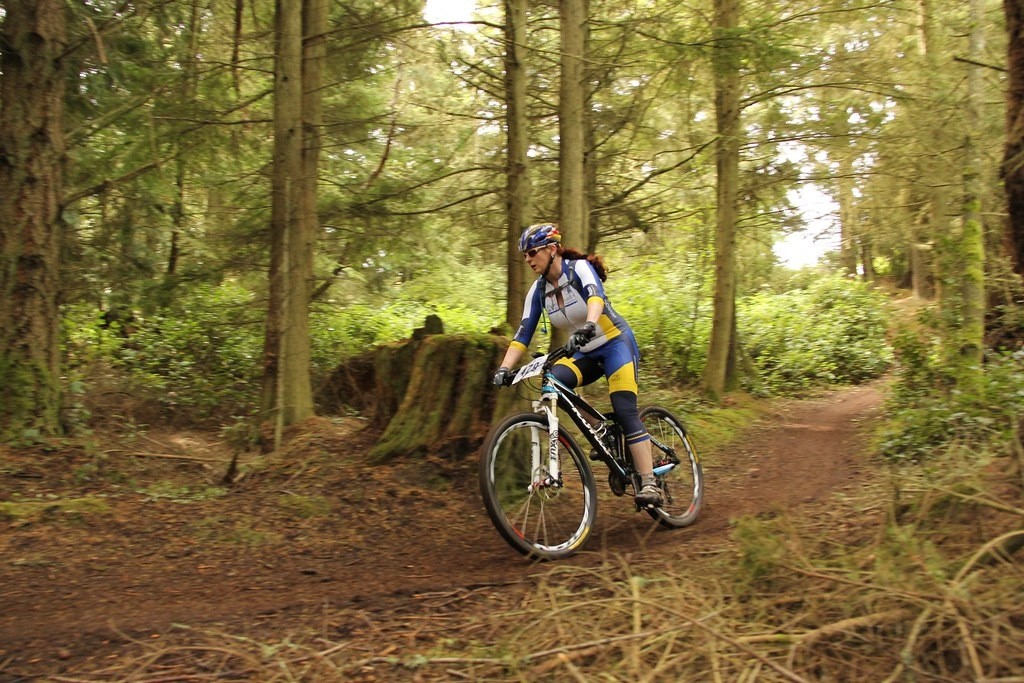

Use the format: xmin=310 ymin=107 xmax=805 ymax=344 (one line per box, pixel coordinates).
xmin=523 ymin=242 xmax=556 ymax=258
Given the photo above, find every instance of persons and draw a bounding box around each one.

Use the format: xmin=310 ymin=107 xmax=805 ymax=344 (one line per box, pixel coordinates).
xmin=493 ymin=223 xmax=664 ymax=509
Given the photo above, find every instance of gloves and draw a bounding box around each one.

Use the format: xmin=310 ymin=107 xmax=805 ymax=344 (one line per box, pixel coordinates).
xmin=572 ymin=322 xmax=597 ymax=346
xmin=492 ymin=367 xmax=510 ymax=387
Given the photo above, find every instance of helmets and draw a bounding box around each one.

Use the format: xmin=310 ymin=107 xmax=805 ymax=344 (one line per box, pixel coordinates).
xmin=517 ymin=223 xmax=561 ymax=252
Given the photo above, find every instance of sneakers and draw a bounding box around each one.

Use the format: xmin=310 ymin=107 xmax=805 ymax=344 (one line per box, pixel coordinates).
xmin=634 ymin=482 xmax=664 ymax=506
xmin=589 ymin=448 xmax=600 ymax=460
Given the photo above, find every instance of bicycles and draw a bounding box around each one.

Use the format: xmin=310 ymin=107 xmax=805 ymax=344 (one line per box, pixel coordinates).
xmin=477 ymin=330 xmax=704 ymax=562
